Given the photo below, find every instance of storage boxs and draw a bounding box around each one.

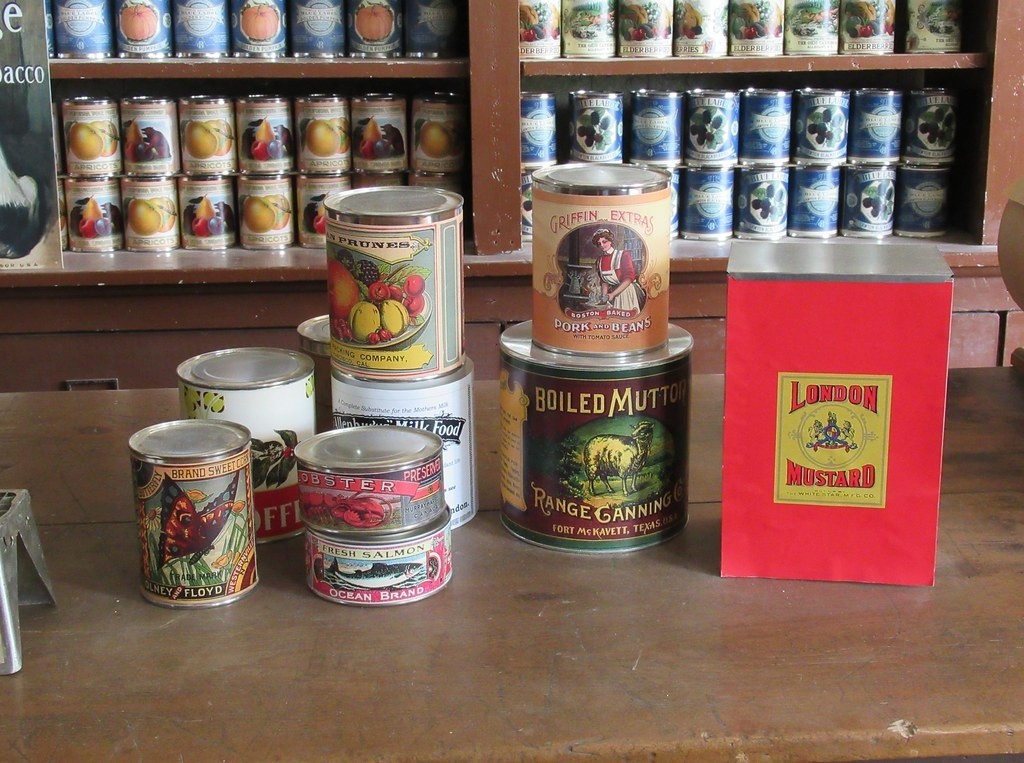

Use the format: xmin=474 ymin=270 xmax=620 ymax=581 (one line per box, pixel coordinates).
xmin=719 ymin=238 xmax=954 ymax=589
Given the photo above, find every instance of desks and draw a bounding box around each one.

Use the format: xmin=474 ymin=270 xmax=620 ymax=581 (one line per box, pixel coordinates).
xmin=0 ymin=366 xmax=1024 ymax=763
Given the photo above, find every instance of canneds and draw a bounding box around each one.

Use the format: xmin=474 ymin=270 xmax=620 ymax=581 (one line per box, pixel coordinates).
xmin=517 ymin=0 xmax=974 ymax=245
xmin=129 ymin=164 xmax=694 ymax=609
xmin=49 ymin=1 xmax=468 ymax=255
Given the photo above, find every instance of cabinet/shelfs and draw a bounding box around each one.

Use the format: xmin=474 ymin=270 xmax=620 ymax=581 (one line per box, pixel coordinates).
xmin=0 ymin=0 xmax=1023 ymax=392
